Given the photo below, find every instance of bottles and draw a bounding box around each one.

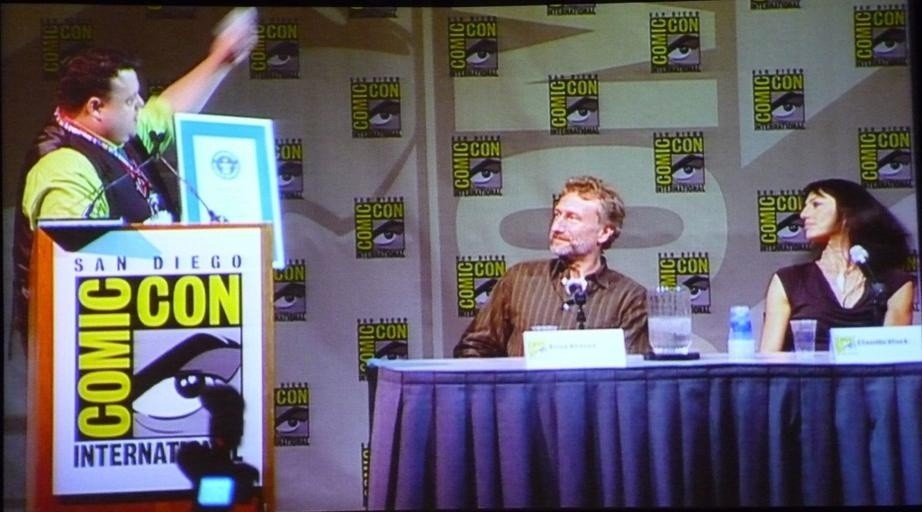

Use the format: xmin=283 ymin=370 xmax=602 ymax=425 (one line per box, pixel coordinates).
xmin=727 ymin=305 xmax=755 ymax=359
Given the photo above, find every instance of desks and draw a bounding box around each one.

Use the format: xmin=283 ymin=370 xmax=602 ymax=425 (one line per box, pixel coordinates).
xmin=367 ymin=359 xmax=921 ymax=509
xmin=34 ymin=227 xmax=275 ymax=512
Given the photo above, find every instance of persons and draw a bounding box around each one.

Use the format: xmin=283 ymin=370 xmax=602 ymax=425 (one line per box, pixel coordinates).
xmin=15 ymin=7 xmax=258 ymax=220
xmin=453 ymin=177 xmax=650 ymax=356
xmin=760 ymin=179 xmax=913 ymax=356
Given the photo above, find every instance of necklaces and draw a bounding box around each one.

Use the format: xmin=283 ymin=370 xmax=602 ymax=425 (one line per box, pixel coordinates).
xmin=820 ymin=257 xmax=857 ymax=292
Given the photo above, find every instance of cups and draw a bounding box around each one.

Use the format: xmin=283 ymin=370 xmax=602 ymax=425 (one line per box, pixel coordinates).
xmin=726 ymin=306 xmax=755 ymax=360
xmin=790 ymin=320 xmax=817 ymax=360
xmin=645 ymin=286 xmax=692 ymax=355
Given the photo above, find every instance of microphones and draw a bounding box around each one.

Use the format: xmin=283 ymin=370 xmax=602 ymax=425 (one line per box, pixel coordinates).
xmin=562 ymin=277 xmax=588 ymax=306
xmin=848 ymin=243 xmax=888 ymax=302
xmin=84 ymin=132 xmax=165 ymax=219
xmin=147 ymin=129 xmax=227 ymax=222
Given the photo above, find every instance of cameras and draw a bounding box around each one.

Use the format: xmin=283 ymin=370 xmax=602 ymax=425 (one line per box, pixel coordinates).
xmin=172 ymin=382 xmax=260 ymax=511
